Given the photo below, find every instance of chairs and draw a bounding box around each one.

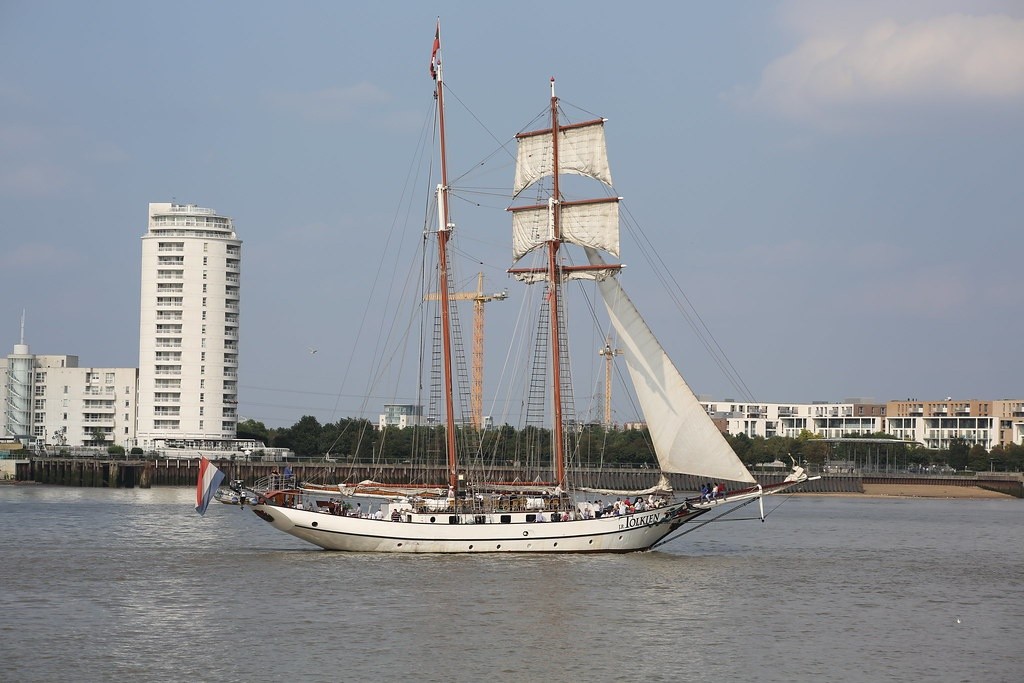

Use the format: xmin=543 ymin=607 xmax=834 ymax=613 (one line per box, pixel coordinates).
xmin=406 ymin=495 xmax=572 ymax=512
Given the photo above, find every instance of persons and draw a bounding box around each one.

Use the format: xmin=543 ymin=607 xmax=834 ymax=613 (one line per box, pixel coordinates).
xmin=552 ymin=495 xmax=666 ymax=521
xmin=391 ymin=509 xmax=402 ymax=522
xmin=823 ymin=462 xmax=855 ymax=474
xmin=287 ymin=497 xmax=313 ymax=511
xmin=271 ymin=467 xmax=280 ymax=490
xmin=700 ymin=482 xmax=728 ymax=505
xmin=907 ymin=462 xmax=967 ymax=476
xmin=375 ymin=508 xmax=384 ymax=520
xmin=328 ymin=497 xmax=362 ymax=518
xmin=284 ymin=463 xmax=296 ymax=489
xmin=541 ymin=489 xmax=572 ymax=510
xmin=408 ymin=492 xmax=426 ymax=512
xmin=461 ymin=490 xmax=527 ymax=511
xmin=536 ymin=509 xmax=544 ymax=523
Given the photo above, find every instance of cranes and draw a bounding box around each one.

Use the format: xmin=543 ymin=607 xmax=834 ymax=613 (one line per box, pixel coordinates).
xmin=424 ymin=270 xmax=509 ymax=431
xmin=600 ymin=334 xmax=624 ymax=432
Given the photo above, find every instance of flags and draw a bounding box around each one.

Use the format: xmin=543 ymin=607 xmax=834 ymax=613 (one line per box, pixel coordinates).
xmin=195 ymin=456 xmax=225 ymax=517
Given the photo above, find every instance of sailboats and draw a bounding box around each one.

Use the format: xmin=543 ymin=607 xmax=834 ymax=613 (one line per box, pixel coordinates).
xmin=214 ymin=15 xmax=820 ymax=552
